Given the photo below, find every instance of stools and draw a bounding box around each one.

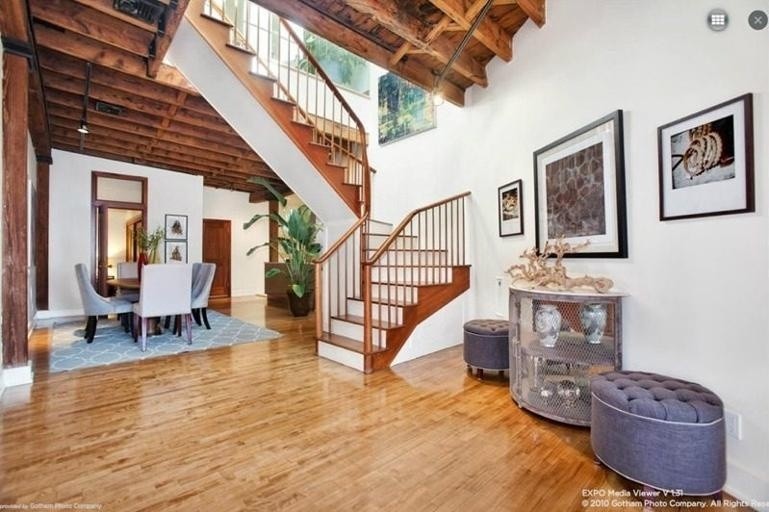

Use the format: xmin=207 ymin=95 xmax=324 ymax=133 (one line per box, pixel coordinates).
xmin=464 ymin=320 xmax=509 ymax=379
xmin=590 ymin=371 xmax=725 ymax=512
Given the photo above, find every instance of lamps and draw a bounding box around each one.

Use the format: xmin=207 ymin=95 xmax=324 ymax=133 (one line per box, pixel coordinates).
xmin=77 ymin=121 xmax=88 ymax=134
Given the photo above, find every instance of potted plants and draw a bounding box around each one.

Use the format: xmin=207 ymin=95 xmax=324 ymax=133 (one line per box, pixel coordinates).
xmin=125 ymin=226 xmax=164 ymax=284
xmin=243 ymin=176 xmax=325 ymax=316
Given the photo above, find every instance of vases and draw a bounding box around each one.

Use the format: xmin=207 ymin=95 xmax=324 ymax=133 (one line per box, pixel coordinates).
xmin=535 ymin=305 xmax=561 ymax=349
xmin=578 ymin=303 xmax=607 ymax=345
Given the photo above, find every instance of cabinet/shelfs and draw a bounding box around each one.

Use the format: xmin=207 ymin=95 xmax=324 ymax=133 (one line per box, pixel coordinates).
xmin=507 ymin=284 xmax=631 ymax=427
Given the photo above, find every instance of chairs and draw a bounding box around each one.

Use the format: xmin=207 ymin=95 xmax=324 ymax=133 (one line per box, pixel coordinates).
xmin=165 ymin=262 xmax=217 ymax=337
xmin=117 ymin=262 xmax=139 ymax=333
xmin=132 ymin=262 xmax=193 ymax=351
xmin=75 ymin=263 xmax=134 ymax=343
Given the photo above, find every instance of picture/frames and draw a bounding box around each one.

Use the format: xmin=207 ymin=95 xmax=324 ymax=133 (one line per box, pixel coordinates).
xmin=165 ymin=214 xmax=188 ymax=240
xmin=498 ymin=179 xmax=523 ymax=237
xmin=376 ymin=74 xmax=436 ymax=147
xmin=533 ymin=110 xmax=628 ymax=258
xmin=165 ymin=241 xmax=188 ymax=264
xmin=657 ymin=93 xmax=754 ymax=220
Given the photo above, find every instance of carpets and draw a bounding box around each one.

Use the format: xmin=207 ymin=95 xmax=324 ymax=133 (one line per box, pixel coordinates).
xmin=49 ymin=308 xmax=284 ymax=376
